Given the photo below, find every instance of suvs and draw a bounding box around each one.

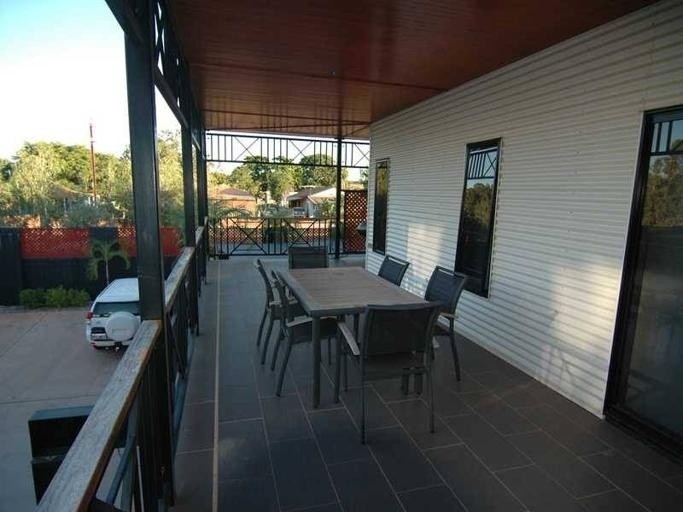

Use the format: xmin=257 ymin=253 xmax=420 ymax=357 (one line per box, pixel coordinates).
xmin=84 ymin=278 xmax=141 ymax=354
xmin=291 ymin=206 xmax=305 ymax=220
